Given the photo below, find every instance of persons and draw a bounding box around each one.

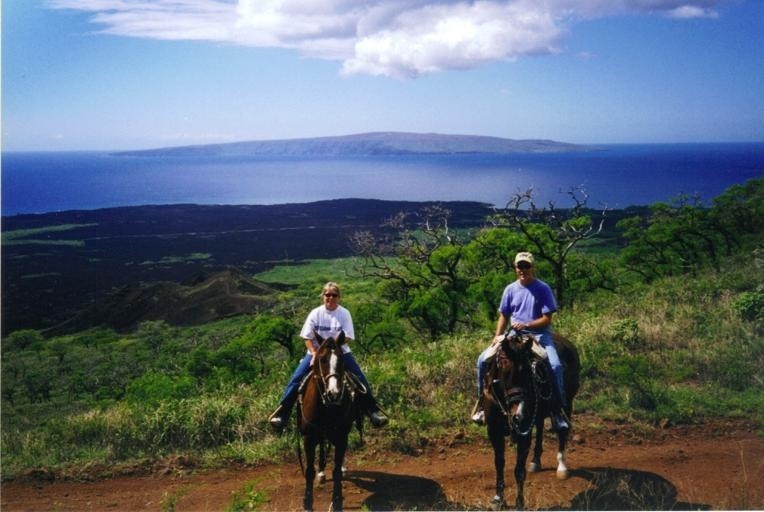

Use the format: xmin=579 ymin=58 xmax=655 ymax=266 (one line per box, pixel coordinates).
xmin=267 ymin=282 xmax=389 ymax=428
xmin=470 ymin=251 xmax=570 ymax=433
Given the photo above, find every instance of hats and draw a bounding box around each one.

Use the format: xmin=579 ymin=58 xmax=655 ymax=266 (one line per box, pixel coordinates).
xmin=514 ymin=252 xmax=534 ymax=266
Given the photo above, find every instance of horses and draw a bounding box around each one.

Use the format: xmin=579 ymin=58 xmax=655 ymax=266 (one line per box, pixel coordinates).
xmin=477 ymin=330 xmax=583 ymax=511
xmin=295 ymin=328 xmax=357 ymax=512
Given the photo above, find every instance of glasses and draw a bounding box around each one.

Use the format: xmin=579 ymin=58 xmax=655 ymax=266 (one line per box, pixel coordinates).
xmin=324 ymin=293 xmax=340 ymax=298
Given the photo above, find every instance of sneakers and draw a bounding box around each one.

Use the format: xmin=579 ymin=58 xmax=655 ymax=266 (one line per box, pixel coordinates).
xmin=270 ymin=417 xmax=284 ymax=433
xmin=556 ymin=419 xmax=569 ymax=429
xmin=472 ymin=410 xmax=486 ymax=427
xmin=372 ymin=416 xmax=390 ymax=429
